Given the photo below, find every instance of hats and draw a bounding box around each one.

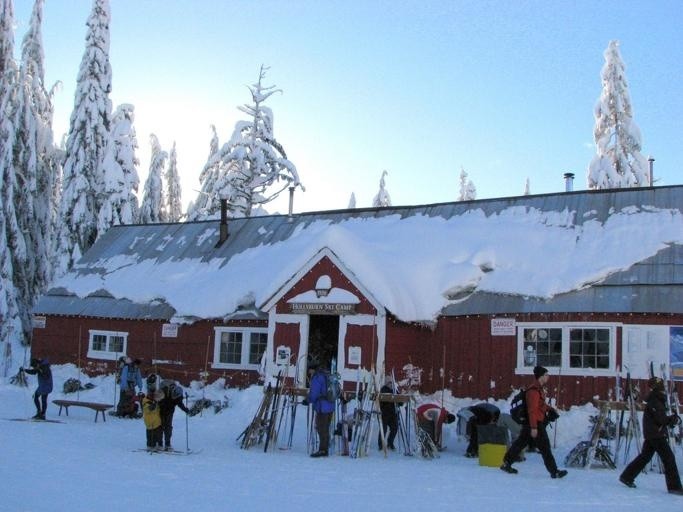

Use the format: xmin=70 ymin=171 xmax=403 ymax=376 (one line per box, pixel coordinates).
xmin=133 ymin=358 xmax=143 ymax=366
xmin=307 ymin=358 xmax=320 ymax=368
xmin=533 ymin=366 xmax=548 ymax=379
xmin=170 ymin=387 xmax=183 ymax=400
xmin=647 ymin=377 xmax=664 ymax=391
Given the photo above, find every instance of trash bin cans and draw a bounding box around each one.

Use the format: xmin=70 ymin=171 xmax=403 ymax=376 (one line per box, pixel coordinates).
xmin=477 ymin=424 xmax=506 ymax=467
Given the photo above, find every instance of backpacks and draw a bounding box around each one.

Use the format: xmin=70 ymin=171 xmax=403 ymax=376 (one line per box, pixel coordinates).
xmin=325 ymin=371 xmax=341 ymax=402
xmin=510 ymin=386 xmax=541 ymax=424
xmin=115 ymin=356 xmax=132 ymax=383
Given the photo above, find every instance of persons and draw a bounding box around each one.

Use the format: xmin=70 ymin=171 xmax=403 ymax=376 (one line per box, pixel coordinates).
xmin=114 ymin=355 xmax=157 ymax=420
xmin=415 ymin=404 xmax=541 ymax=459
xmin=141 ymin=387 xmax=166 ymax=455
xmin=619 ymin=376 xmax=683 ymax=495
xmin=157 ymin=381 xmax=195 ymax=452
xmin=499 ymin=366 xmax=568 ymax=479
xmin=19 ymin=358 xmax=53 ymax=421
xmin=302 ymin=359 xmax=335 ymax=458
xmin=378 ymin=375 xmax=399 ymax=451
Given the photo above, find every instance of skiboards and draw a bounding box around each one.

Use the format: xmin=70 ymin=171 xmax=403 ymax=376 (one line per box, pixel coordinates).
xmin=237 ymin=352 xmax=321 ymax=458
xmin=131 ymin=446 xmax=203 ymax=456
xmin=603 ymin=362 xmax=680 ymax=475
xmin=6 ymin=417 xmax=65 ymax=423
xmin=330 ymin=358 xmax=440 ymax=461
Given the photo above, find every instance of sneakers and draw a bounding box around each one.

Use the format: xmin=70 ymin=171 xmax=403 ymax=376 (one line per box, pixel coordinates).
xmin=551 ymin=470 xmax=568 ymax=478
xmin=668 ymin=488 xmax=683 ymax=495
xmin=311 ymin=450 xmax=327 ymax=457
xmin=147 ymin=444 xmax=173 ymax=453
xmin=500 ymin=465 xmax=517 ymax=473
xmin=620 ymin=475 xmax=636 ymax=488
xmin=32 ymin=413 xmax=45 ymax=420
xmin=387 ymin=439 xmax=395 ymax=449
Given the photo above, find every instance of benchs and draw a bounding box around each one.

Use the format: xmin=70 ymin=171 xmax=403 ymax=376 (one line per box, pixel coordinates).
xmin=52 ymin=399 xmax=114 ymax=422
